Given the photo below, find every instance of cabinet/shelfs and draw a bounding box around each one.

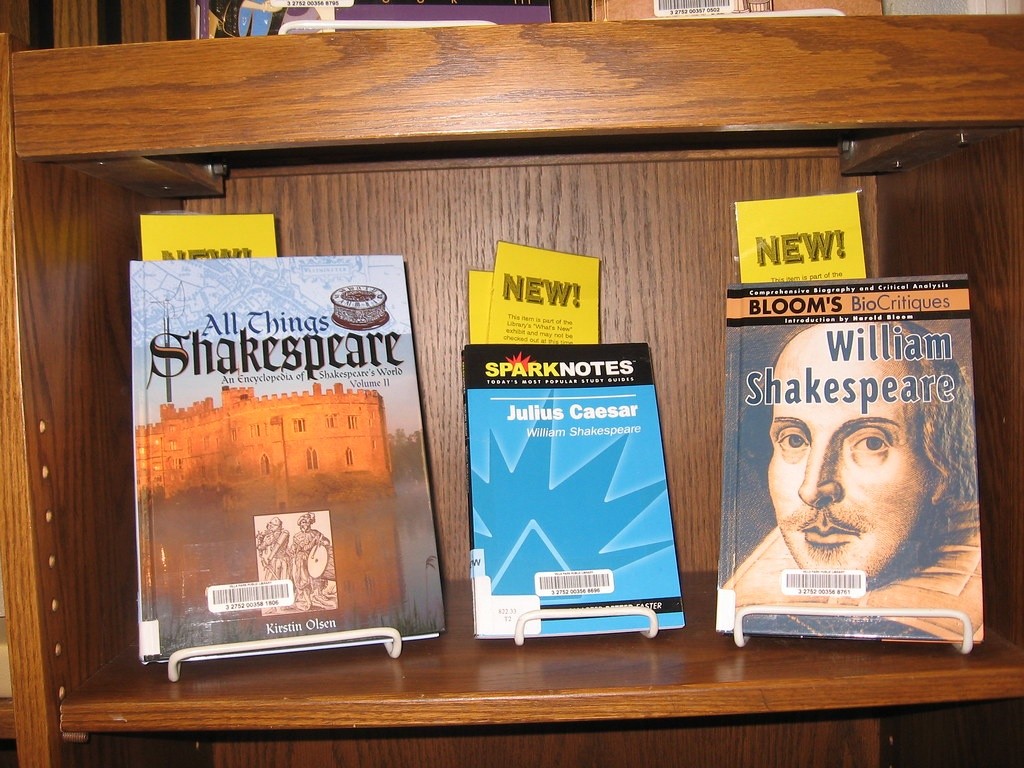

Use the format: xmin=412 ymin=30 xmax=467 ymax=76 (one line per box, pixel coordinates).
xmin=1 ymin=0 xmax=1024 ymax=767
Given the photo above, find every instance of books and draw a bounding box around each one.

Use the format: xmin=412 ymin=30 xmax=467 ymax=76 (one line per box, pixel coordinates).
xmin=129 ymin=256 xmax=448 ymax=665
xmin=715 ymin=271 xmax=986 ymax=640
xmin=460 ymin=339 xmax=688 ymax=641
